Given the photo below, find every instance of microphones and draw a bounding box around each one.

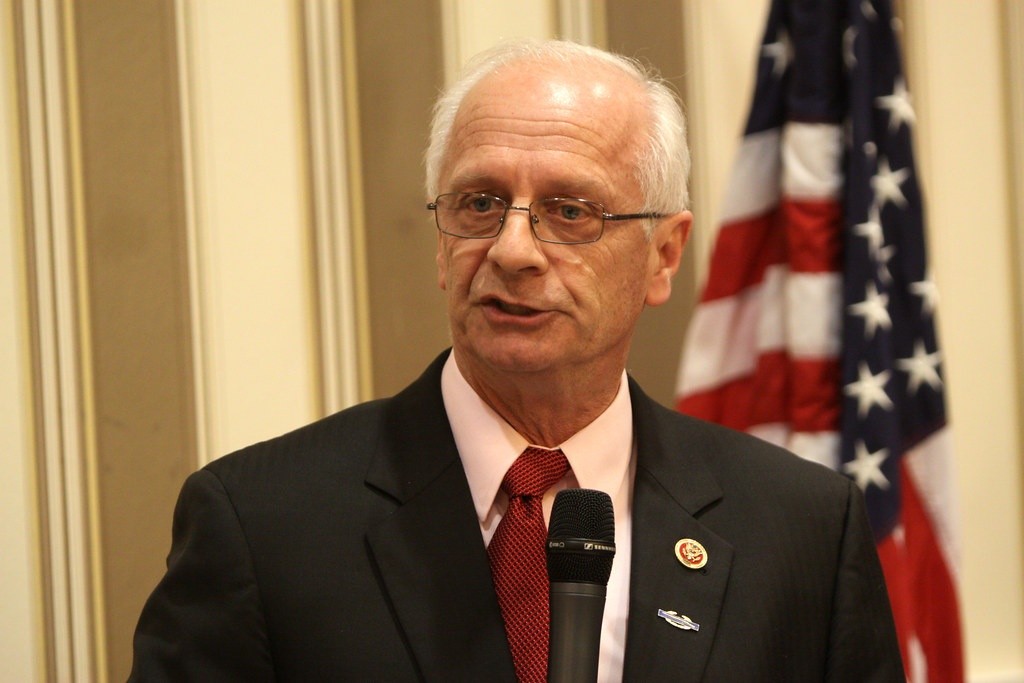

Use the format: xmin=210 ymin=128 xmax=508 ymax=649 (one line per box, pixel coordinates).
xmin=544 ymin=490 xmax=615 ymax=683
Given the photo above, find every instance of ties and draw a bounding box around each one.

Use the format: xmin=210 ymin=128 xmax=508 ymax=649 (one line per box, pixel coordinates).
xmin=485 ymin=446 xmax=571 ymax=683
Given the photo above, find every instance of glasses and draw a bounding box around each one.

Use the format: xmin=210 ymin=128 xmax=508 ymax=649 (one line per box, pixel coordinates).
xmin=425 ymin=192 xmax=667 ymax=245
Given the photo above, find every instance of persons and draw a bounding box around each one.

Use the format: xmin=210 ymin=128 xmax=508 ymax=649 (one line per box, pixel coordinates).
xmin=123 ymin=38 xmax=908 ymax=683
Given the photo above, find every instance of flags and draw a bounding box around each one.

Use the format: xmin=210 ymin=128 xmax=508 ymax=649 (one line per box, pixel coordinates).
xmin=670 ymin=0 xmax=966 ymax=683
